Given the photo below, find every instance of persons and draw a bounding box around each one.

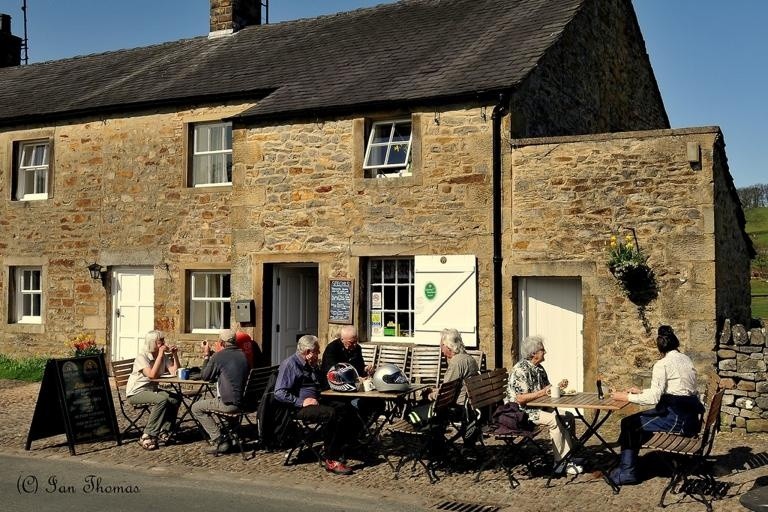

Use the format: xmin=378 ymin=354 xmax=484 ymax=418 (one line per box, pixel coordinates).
xmin=610 ymin=325 xmax=706 ymax=486
xmin=507 ymin=336 xmax=584 ymax=474
xmin=126 ymin=330 xmax=182 ymax=451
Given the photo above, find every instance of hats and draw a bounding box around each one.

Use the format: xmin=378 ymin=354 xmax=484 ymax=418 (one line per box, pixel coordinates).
xmin=220 ymin=329 xmax=237 ymax=342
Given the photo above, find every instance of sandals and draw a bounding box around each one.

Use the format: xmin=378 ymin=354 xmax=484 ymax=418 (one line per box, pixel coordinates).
xmin=157 ymin=429 xmax=181 ymax=445
xmin=138 ymin=433 xmax=159 ymax=451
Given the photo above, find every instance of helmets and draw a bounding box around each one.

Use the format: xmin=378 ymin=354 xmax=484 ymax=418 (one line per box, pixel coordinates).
xmin=326 ymin=362 xmax=361 ymax=393
xmin=372 ymin=362 xmax=411 ymax=394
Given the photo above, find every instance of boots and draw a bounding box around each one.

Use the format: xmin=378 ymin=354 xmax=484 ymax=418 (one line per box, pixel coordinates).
xmin=605 ymin=448 xmax=639 ymax=486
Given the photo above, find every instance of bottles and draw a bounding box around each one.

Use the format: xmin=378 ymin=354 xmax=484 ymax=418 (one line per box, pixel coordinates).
xmin=596 ymin=379 xmax=609 ymax=400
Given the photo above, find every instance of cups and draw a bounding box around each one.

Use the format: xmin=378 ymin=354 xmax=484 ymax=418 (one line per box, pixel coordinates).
xmin=546 ymin=385 xmax=561 ymax=399
xmin=363 ymin=379 xmax=373 ymax=391
xmin=177 ymin=367 xmax=190 ymax=380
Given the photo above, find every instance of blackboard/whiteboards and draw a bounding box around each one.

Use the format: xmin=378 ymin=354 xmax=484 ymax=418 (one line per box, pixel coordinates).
xmin=327 ymin=277 xmax=355 ymax=324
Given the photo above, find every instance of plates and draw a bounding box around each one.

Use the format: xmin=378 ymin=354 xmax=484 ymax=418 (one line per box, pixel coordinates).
xmin=159 ymin=374 xmax=177 ymax=378
xmin=558 ymin=393 xmax=579 ymax=395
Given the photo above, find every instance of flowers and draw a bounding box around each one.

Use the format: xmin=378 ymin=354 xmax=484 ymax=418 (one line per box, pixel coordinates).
xmin=393 ymin=141 xmax=412 ymax=163
xmin=604 ymin=234 xmax=648 ymax=298
xmin=60 ymin=333 xmax=104 ymax=359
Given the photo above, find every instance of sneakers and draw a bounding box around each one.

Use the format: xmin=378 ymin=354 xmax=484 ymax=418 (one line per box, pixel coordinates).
xmin=206 ymin=438 xmax=232 ymax=455
xmin=553 ymin=460 xmax=584 ymax=475
xmin=325 ymin=459 xmax=354 ymax=475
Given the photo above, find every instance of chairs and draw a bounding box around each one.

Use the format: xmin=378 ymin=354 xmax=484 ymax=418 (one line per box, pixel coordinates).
xmin=106 ymin=337 xmax=729 ymax=512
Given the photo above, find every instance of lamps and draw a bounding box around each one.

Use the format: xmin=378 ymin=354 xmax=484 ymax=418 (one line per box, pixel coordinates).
xmin=86 ymin=259 xmax=106 ymax=287
